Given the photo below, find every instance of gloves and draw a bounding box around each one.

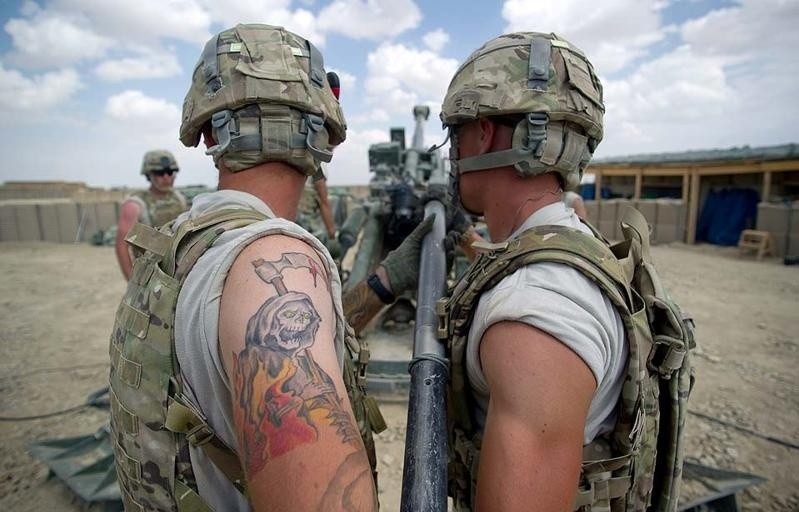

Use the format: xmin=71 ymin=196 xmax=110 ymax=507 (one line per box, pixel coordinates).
xmin=382 ymin=214 xmax=463 ymax=295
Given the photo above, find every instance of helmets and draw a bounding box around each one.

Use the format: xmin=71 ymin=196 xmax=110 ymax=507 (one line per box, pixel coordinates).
xmin=440 ymin=31 xmax=606 ymax=190
xmin=180 ymin=23 xmax=347 ymax=175
xmin=140 ymin=150 xmax=179 ymax=178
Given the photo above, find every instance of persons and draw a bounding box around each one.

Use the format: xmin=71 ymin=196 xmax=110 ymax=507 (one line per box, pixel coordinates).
xmin=294 ymin=161 xmax=336 ymax=247
xmin=559 ymin=188 xmax=588 ymax=220
xmin=106 ymin=23 xmax=462 ymax=511
xmin=115 ymin=149 xmax=187 ymax=281
xmin=408 ymin=28 xmax=646 ymax=511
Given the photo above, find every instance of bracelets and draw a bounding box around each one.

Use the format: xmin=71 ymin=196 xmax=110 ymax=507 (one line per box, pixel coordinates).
xmin=367 ymin=274 xmax=396 ymax=304
